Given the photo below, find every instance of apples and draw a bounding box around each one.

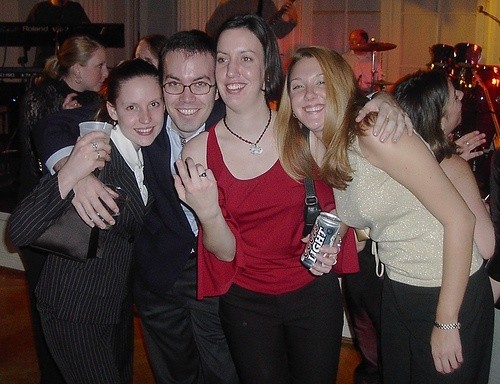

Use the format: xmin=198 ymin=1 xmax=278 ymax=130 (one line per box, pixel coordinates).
xmin=349 ymin=29 xmax=368 ymax=45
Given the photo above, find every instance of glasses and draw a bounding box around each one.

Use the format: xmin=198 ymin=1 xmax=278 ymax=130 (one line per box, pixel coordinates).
xmin=162 ymin=81 xmax=218 ymax=95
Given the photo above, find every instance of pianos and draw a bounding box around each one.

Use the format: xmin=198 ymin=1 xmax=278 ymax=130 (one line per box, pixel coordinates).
xmin=0 ymin=22 xmax=125 ymax=49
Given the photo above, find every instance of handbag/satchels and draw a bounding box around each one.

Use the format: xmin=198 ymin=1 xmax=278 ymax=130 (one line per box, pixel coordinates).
xmin=29 ymin=169 xmax=114 ymax=264
xmin=303 ymin=175 xmax=321 ymax=237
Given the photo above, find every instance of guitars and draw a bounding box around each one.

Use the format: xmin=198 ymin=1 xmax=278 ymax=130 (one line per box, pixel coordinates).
xmin=266 ymin=0 xmax=294 ymax=27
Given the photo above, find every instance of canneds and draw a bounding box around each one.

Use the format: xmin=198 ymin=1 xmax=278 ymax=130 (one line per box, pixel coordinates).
xmin=300 ymin=212 xmax=341 ymax=269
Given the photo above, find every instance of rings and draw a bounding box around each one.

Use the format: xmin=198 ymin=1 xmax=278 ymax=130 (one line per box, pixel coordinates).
xmin=199 ymin=173 xmax=207 ymax=178
xmin=466 ymin=141 xmax=470 ymax=148
xmin=92 ymin=142 xmax=98 ymax=150
xmin=97 ymin=150 xmax=102 ymax=160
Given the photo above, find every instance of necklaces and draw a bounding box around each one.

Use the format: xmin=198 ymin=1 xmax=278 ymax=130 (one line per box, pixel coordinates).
xmin=223 ymin=108 xmax=272 ymax=155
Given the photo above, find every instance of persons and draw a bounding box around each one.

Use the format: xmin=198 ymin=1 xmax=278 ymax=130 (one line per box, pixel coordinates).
xmin=136 ymin=33 xmax=168 ymax=71
xmin=390 ymin=67 xmax=496 ymax=259
xmin=275 ymin=45 xmax=495 ymax=384
xmin=342 ymin=29 xmax=383 ymax=94
xmin=32 ymin=29 xmax=413 ymax=384
xmin=24 ymin=0 xmax=91 ymax=69
xmin=205 ymin=0 xmax=298 ymax=40
xmin=173 ymin=15 xmax=358 ymax=384
xmin=342 ymin=232 xmax=382 ymax=384
xmin=11 ymin=37 xmax=108 ymax=383
xmin=7 ymin=59 xmax=165 ymax=384
xmin=484 ymin=100 xmax=500 ymax=384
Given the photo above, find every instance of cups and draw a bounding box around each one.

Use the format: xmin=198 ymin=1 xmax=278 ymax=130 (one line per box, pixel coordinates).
xmin=79 ymin=122 xmax=113 ymax=162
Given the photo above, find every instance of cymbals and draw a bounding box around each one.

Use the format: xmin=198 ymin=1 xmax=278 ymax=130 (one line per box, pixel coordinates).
xmin=365 ymin=80 xmax=393 ymax=85
xmin=350 ymin=41 xmax=397 ymax=52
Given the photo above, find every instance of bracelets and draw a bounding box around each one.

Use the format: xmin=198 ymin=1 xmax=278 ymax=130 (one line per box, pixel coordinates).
xmin=434 ymin=321 xmax=461 ymax=330
xmin=337 ymin=231 xmax=343 ymax=243
